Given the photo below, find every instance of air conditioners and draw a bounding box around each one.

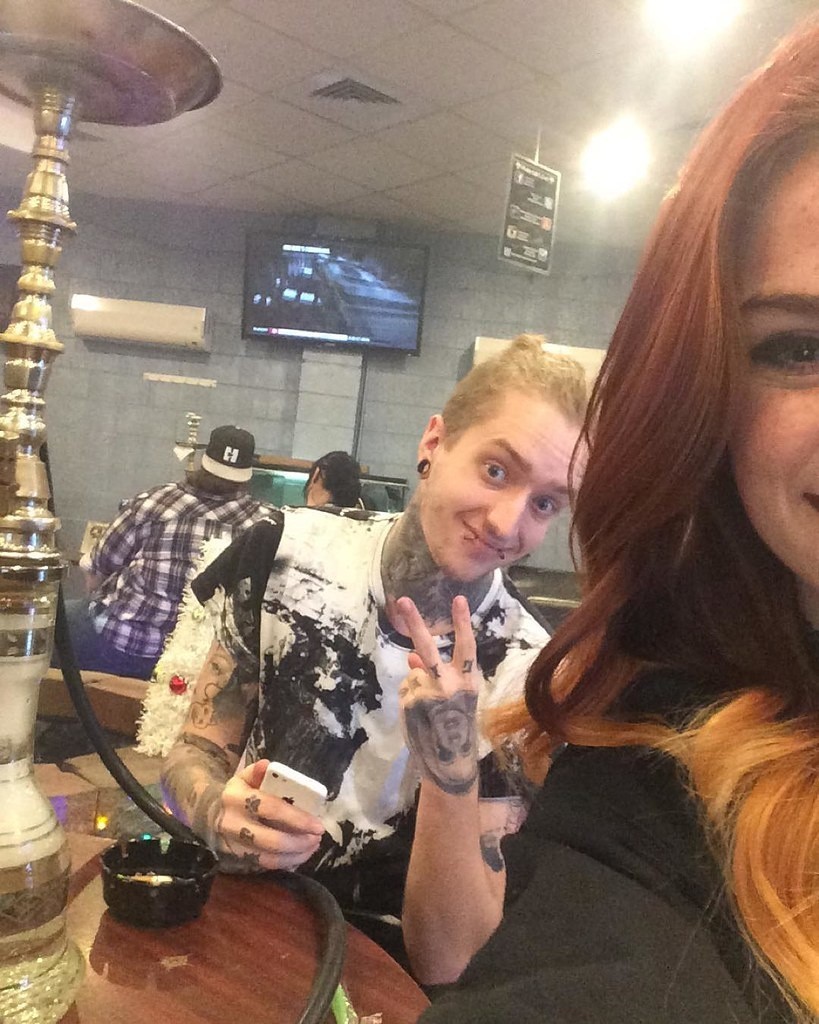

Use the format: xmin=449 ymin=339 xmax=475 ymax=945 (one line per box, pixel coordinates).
xmin=71 ymin=294 xmax=213 ymax=355
xmin=474 ymin=337 xmax=607 ymax=381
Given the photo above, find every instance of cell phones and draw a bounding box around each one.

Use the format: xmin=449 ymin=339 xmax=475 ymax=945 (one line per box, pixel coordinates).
xmin=259 ymin=761 xmax=327 ymax=817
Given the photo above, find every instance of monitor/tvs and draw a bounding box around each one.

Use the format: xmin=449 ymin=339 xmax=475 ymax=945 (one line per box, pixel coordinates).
xmin=241 ymin=230 xmax=430 ymax=358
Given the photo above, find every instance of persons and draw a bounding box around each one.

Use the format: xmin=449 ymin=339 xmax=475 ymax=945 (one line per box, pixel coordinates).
xmin=75 ymin=425 xmax=281 ymax=676
xmin=305 ymin=452 xmax=365 ymax=510
xmin=415 ymin=6 xmax=819 ymax=1024
xmin=165 ymin=331 xmax=606 ymax=989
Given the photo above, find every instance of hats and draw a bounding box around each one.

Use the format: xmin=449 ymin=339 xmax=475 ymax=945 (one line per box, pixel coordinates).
xmin=201 ymin=425 xmax=255 ymax=482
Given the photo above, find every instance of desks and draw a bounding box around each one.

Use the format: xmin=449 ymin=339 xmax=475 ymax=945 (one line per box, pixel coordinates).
xmin=51 ymin=834 xmax=431 ymax=1024
